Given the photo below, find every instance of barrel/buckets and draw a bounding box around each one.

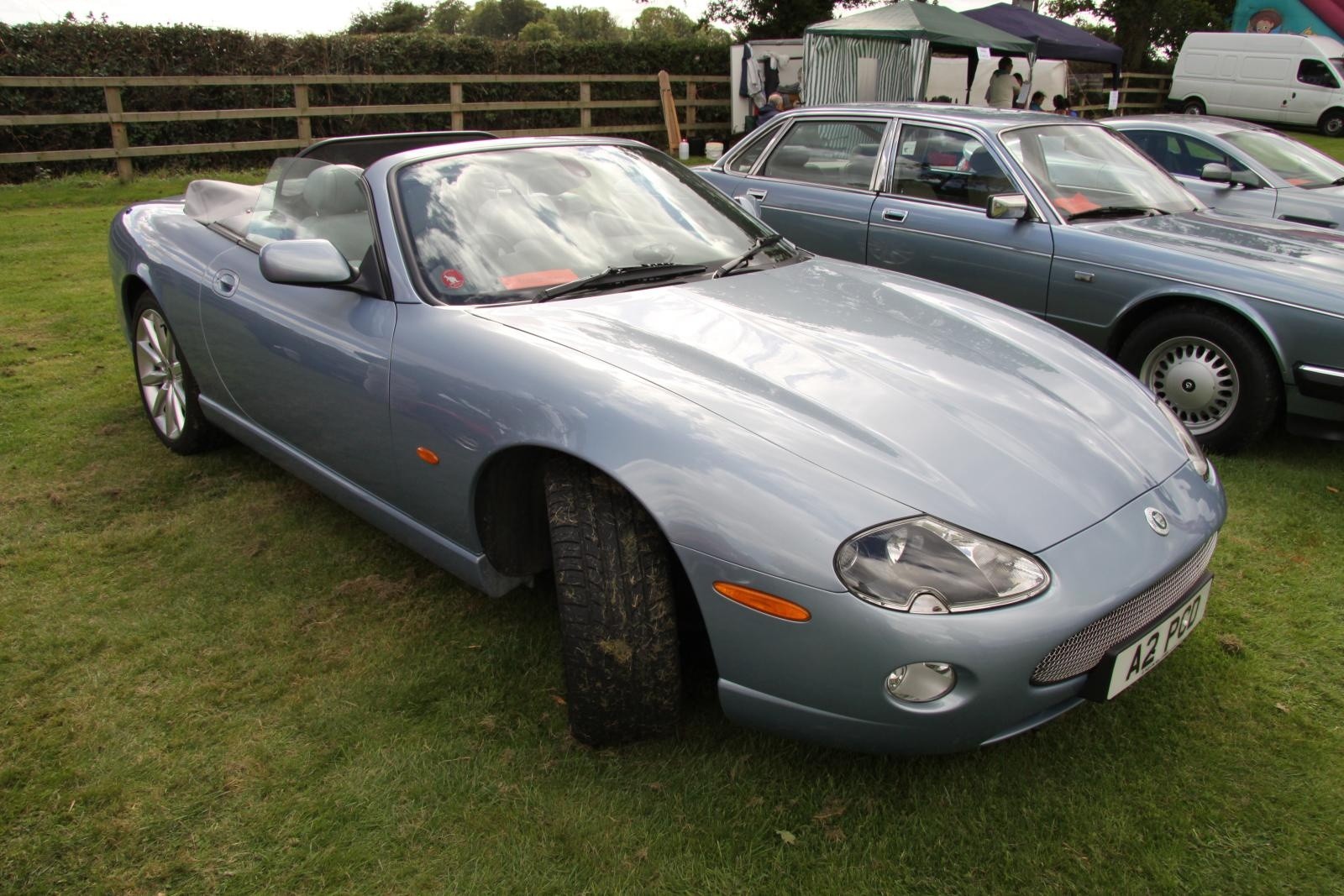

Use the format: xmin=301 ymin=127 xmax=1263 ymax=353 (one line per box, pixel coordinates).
xmin=705 ymin=142 xmax=724 ymax=161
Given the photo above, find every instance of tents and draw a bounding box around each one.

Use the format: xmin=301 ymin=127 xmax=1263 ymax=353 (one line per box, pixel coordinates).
xmin=931 ymin=2 xmax=1123 ymax=117
xmin=801 ymin=0 xmax=1037 ymax=110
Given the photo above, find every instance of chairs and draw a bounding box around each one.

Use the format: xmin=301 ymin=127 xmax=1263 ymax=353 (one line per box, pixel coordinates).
xmin=841 ymin=143 xmax=880 ymax=189
xmin=1132 ymin=130 xmax=1180 ymax=172
xmin=899 ymin=160 xmax=938 ymax=201
xmin=775 ymin=145 xmax=825 ymax=184
xmin=301 ymin=163 xmax=376 ymax=263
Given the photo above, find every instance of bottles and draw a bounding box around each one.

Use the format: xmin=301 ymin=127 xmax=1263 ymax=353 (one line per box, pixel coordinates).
xmin=679 ymin=139 xmax=689 ymax=160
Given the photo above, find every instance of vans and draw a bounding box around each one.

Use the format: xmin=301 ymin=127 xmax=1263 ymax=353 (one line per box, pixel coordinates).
xmin=1166 ymin=31 xmax=1344 ymax=138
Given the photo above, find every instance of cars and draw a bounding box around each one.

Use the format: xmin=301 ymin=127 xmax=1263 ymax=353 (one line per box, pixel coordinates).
xmin=683 ymin=99 xmax=1344 ymax=459
xmin=977 ymin=112 xmax=1344 ymax=232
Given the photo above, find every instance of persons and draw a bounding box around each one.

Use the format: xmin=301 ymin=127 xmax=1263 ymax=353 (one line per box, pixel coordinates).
xmin=793 ymin=66 xmax=804 ymax=109
xmin=1052 ymin=95 xmax=1069 ymax=115
xmin=1012 ymin=72 xmax=1023 ymax=110
xmin=756 ymin=93 xmax=784 ymax=128
xmin=1029 ymin=92 xmax=1045 ymax=111
xmin=989 ymin=57 xmax=1020 ymax=109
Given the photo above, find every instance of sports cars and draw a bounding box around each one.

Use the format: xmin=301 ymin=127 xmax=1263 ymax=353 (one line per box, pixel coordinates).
xmin=107 ymin=124 xmax=1230 ymax=757
